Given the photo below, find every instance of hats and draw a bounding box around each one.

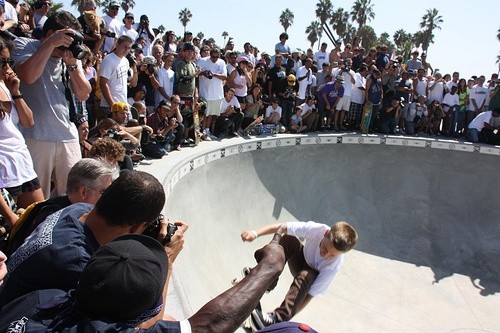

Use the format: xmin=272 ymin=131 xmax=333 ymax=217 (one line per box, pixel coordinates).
xmin=109 ymin=1 xmax=120 ymax=7
xmin=125 ymin=12 xmax=134 ymax=18
xmin=137 ymin=56 xmax=157 ymax=66
xmin=131 ymin=43 xmax=144 ymax=54
xmin=254 ymin=321 xmax=319 ymax=333
xmin=288 ymin=74 xmax=296 ymax=86
xmin=107 ymin=101 xmax=129 ymax=117
xmin=159 ymin=99 xmax=172 ymax=111
xmin=69 ymin=112 xmax=88 ymax=127
xmin=257 ymin=59 xmax=266 ymax=65
xmin=334 ymin=75 xmax=345 ymax=83
xmin=77 ymin=234 xmax=169 ymax=329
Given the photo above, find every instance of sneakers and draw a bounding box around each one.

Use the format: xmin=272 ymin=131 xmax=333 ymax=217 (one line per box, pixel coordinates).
xmin=251 ymin=308 xmax=282 ymax=331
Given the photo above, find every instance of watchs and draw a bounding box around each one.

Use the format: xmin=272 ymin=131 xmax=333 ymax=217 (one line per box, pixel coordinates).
xmin=67 ymin=64 xmax=78 ymax=72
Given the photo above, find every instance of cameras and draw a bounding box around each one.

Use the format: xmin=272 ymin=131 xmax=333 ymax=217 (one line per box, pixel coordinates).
xmin=57 ymin=32 xmax=88 ymax=60
xmin=143 ymin=215 xmax=178 ymax=247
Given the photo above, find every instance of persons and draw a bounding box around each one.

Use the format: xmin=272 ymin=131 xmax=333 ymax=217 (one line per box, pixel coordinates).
xmin=0 ymin=0 xmax=500 ymax=333
xmin=99 ymin=35 xmax=132 ymax=118
xmin=0 ymin=158 xmax=117 ymax=257
xmin=197 ymin=48 xmax=227 ymax=141
xmin=0 ymin=224 xmax=286 ymax=333
xmin=231 ymin=220 xmax=358 ymax=333
xmin=13 ymin=11 xmax=92 ymax=201
xmin=0 ymin=170 xmax=189 ymax=329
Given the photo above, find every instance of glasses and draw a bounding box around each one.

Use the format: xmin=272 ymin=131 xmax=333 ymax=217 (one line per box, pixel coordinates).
xmin=0 ymin=60 xmax=14 ymax=68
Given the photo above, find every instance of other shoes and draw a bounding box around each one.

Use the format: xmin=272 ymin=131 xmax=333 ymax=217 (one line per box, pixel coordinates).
xmin=201 ymin=131 xmax=217 ymax=141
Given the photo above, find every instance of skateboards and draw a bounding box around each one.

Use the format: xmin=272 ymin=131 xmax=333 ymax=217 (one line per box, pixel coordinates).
xmin=242 ymin=116 xmax=263 ymax=136
xmin=231 ymin=267 xmax=263 ymax=331
xmin=192 ymin=103 xmax=203 ymax=145
xmin=359 ymin=102 xmax=374 ymax=134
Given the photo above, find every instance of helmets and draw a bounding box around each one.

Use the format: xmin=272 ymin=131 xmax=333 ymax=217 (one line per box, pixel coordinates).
xmin=184 ymin=41 xmax=196 ymax=51
xmin=234 ymin=55 xmax=248 ymax=64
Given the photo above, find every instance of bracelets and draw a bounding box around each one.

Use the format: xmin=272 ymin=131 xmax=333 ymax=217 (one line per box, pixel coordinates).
xmin=11 ymin=94 xmax=23 ymax=100
xmin=141 ymin=124 xmax=146 ymax=131
xmin=149 ymin=73 xmax=154 ymax=78
